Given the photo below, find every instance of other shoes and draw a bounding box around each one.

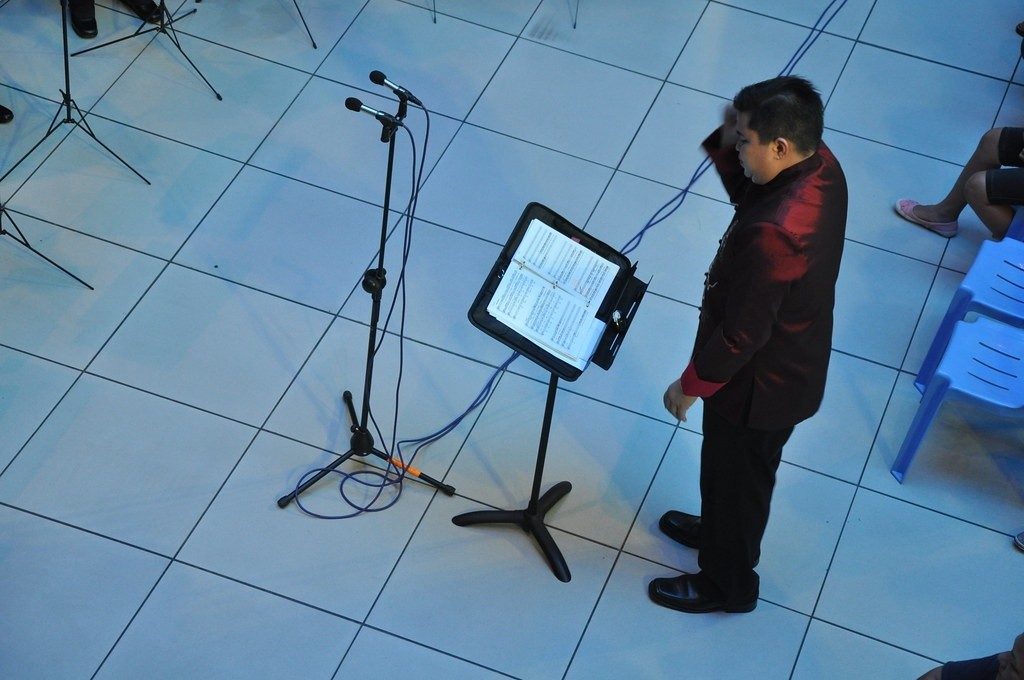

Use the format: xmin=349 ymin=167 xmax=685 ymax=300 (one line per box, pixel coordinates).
xmin=896 ymin=198 xmax=959 ymax=238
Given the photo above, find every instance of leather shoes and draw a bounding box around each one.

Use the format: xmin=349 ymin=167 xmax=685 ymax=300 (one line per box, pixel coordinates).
xmin=648 ymin=572 xmax=759 ymax=614
xmin=660 ymin=510 xmax=761 ymax=569
xmin=68 ymin=0 xmax=98 ymax=38
xmin=0 ymin=105 xmax=13 ymax=125
xmin=121 ymin=0 xmax=162 ymax=23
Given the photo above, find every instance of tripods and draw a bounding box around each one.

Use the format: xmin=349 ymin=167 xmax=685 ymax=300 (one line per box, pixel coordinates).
xmin=276 ymin=129 xmax=457 ymax=510
xmin=449 ymin=372 xmax=571 ymax=582
xmin=1 ymin=0 xmax=222 ymax=291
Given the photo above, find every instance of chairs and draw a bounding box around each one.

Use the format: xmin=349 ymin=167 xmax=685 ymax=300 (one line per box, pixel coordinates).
xmin=914 ymin=208 xmax=1024 ymax=393
xmin=890 ymin=317 xmax=1024 ymax=487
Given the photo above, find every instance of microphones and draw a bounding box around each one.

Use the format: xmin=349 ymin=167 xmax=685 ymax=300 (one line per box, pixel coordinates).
xmin=345 ymin=70 xmax=423 ymax=128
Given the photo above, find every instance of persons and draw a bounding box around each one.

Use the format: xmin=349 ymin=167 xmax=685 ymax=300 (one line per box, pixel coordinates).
xmin=644 ymin=75 xmax=852 ymax=616
xmin=894 ymin=126 xmax=1024 ymax=243
